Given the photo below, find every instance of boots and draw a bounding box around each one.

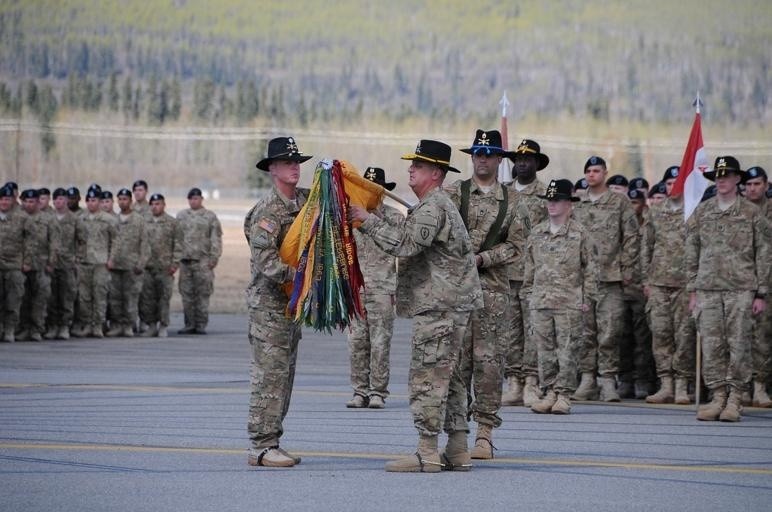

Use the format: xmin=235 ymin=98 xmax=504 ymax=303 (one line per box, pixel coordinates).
xmin=384 ymin=436 xmax=441 ymax=472
xmin=439 ymin=432 xmax=472 ymax=471
xmin=248 ymin=447 xmax=299 ymax=466
xmin=501 ymin=374 xmax=771 ymax=423
xmin=469 ymin=422 xmax=497 ymax=459
xmin=346 ymin=393 xmax=368 ymax=408
xmin=1 ymin=322 xmax=167 ymax=337
xmin=178 ymin=324 xmax=207 ymax=334
xmin=368 ymin=395 xmax=385 ymax=408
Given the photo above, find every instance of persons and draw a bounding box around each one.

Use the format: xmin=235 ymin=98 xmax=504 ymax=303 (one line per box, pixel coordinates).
xmin=498 ymin=136 xmax=772 ymax=425
xmin=345 ymin=168 xmax=406 ymax=411
xmin=241 ymin=136 xmax=316 ymax=468
xmin=344 ymin=137 xmax=489 ymax=473
xmin=1 ymin=178 xmax=224 ymax=342
xmin=442 ymin=130 xmax=533 ymax=461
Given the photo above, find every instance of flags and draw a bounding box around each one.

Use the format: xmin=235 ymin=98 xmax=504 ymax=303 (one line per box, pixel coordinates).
xmin=669 ymin=112 xmax=712 ymax=220
xmin=498 ymin=119 xmax=514 ymax=182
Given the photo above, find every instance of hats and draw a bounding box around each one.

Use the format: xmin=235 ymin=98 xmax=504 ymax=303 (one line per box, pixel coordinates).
xmin=0 ymin=180 xmax=79 ymax=198
xmin=536 ymin=156 xmax=680 ymax=202
xmin=703 ymin=156 xmax=772 ymax=199
xmin=188 ymin=188 xmax=201 ymax=197
xmin=508 ymin=138 xmax=549 ymax=171
xmin=460 ymin=129 xmax=508 ymax=158
xmin=364 ymin=167 xmax=396 ymax=190
xmin=86 ymin=181 xmax=164 ymax=201
xmin=256 ymin=137 xmax=312 ymax=171
xmin=400 ymin=140 xmax=461 ymax=173
